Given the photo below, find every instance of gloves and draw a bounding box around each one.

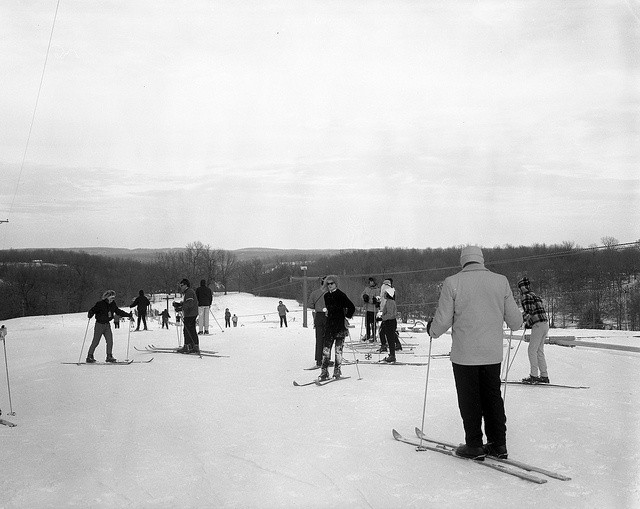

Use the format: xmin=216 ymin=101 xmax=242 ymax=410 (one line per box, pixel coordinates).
xmin=173 ymin=301 xmax=181 ymax=307
xmin=427 ymin=320 xmax=433 ymax=336
xmin=373 ymin=296 xmax=377 ymax=304
xmin=175 ymin=307 xmax=182 ymax=311
xmin=363 ymin=294 xmax=369 ymax=303
xmin=376 ymin=302 xmax=380 ymax=308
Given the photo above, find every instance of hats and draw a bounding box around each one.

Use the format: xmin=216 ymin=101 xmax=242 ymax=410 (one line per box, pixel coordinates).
xmin=460 ymin=246 xmax=486 ymax=268
xmin=386 ymin=287 xmax=395 ymax=297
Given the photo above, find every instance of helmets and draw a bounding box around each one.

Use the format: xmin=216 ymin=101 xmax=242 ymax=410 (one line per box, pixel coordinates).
xmin=102 ymin=290 xmax=116 ymax=301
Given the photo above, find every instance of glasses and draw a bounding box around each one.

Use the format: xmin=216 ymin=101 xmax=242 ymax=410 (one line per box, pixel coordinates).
xmin=181 ymin=285 xmax=183 ymax=286
xmin=326 ymin=282 xmax=335 ymax=285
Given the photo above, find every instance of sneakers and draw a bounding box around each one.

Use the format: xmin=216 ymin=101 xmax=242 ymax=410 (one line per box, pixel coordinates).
xmin=368 ymin=337 xmax=377 ymax=342
xmin=540 ymin=376 xmax=550 ymax=385
xmin=106 ymin=354 xmax=116 ymax=362
xmin=332 ymin=368 xmax=342 ymax=382
xmin=483 ymin=442 xmax=508 ymax=460
xmin=379 ymin=345 xmax=387 ymax=353
xmin=316 ymin=360 xmax=322 ymax=366
xmin=522 ymin=375 xmax=539 ymax=384
xmin=86 ymin=354 xmax=96 ymax=362
xmin=327 ymin=361 xmax=335 ymax=366
xmin=384 ymin=354 xmax=396 ymax=362
xmin=204 ymin=330 xmax=209 ymax=334
xmin=455 ymin=443 xmax=487 ymax=461
xmin=177 ymin=344 xmax=199 ymax=354
xmin=361 ymin=335 xmax=371 ymax=341
xmin=318 ymin=369 xmax=329 ymax=382
xmin=394 ymin=342 xmax=402 ymax=351
xmin=198 ymin=331 xmax=203 ymax=335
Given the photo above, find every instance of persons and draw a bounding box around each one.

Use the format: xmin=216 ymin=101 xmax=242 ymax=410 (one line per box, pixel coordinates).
xmin=113 ymin=314 xmax=122 ymax=328
xmin=361 ymin=277 xmax=381 ymax=342
xmin=176 ymin=314 xmax=180 ymax=326
xmin=308 ymin=276 xmax=334 ymax=366
xmin=375 ymin=279 xmax=402 ymax=350
xmin=196 ymin=280 xmax=212 ymax=335
xmin=158 ymin=309 xmax=171 ymax=329
xmin=232 ymin=314 xmax=238 ymax=327
xmin=172 ymin=279 xmax=199 ymax=354
xmin=86 ymin=290 xmax=132 ymax=362
xmin=518 ymin=277 xmax=549 ymax=384
xmin=318 ymin=274 xmax=355 ymax=378
xmin=127 ymin=312 xmax=135 ymax=328
xmin=376 ymin=288 xmax=397 ymax=362
xmin=225 ymin=308 xmax=231 ymax=327
xmin=427 ymin=246 xmax=524 ymax=459
xmin=277 ymin=301 xmax=289 ymax=327
xmin=130 ymin=290 xmax=150 ymax=332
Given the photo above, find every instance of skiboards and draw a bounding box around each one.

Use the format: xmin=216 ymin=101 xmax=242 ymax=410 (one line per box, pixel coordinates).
xmin=133 ymin=344 xmax=229 ymax=358
xmin=344 ymin=340 xmax=419 ymax=354
xmin=415 ymin=354 xmax=452 ymax=359
xmin=196 ymin=332 xmax=216 ymax=336
xmin=393 ymin=428 xmax=572 ymax=483
xmin=129 ymin=329 xmax=153 ymax=332
xmin=501 ymin=379 xmax=591 ymax=388
xmin=304 ymin=362 xmax=351 ymax=370
xmin=342 ymin=358 xmax=429 ymax=366
xmin=0 ymin=417 xmax=18 ymax=428
xmin=292 ymin=376 xmax=351 ymax=386
xmin=60 ymin=357 xmax=154 ymax=365
